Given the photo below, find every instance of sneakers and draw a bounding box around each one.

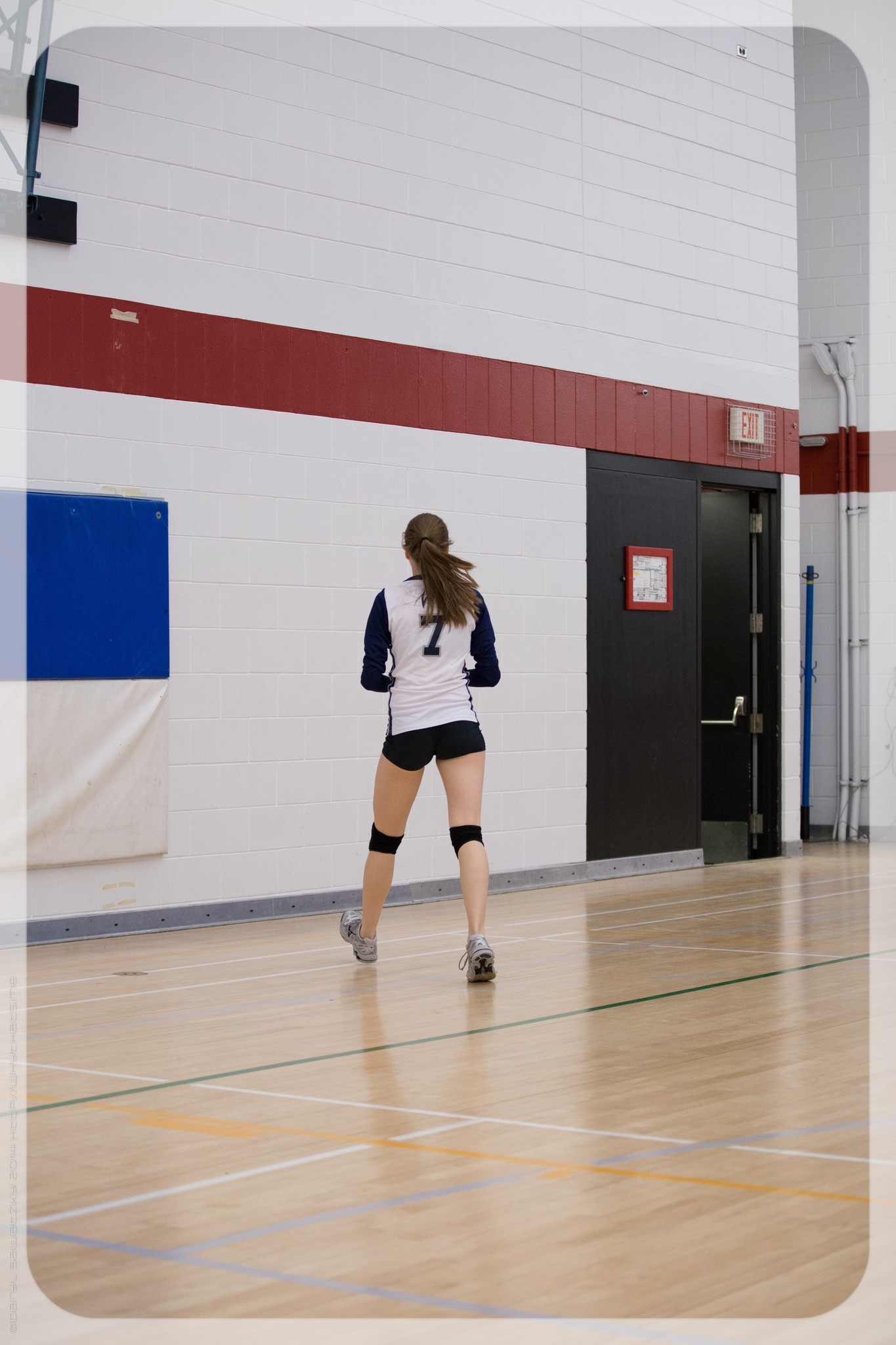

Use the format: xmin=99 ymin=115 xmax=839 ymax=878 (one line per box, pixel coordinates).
xmin=458 ymin=934 xmax=496 ymax=983
xmin=339 ymin=909 xmax=378 ymax=963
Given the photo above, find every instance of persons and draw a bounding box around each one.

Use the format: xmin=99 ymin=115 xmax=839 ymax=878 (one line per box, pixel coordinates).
xmin=339 ymin=512 xmax=502 ymax=982
